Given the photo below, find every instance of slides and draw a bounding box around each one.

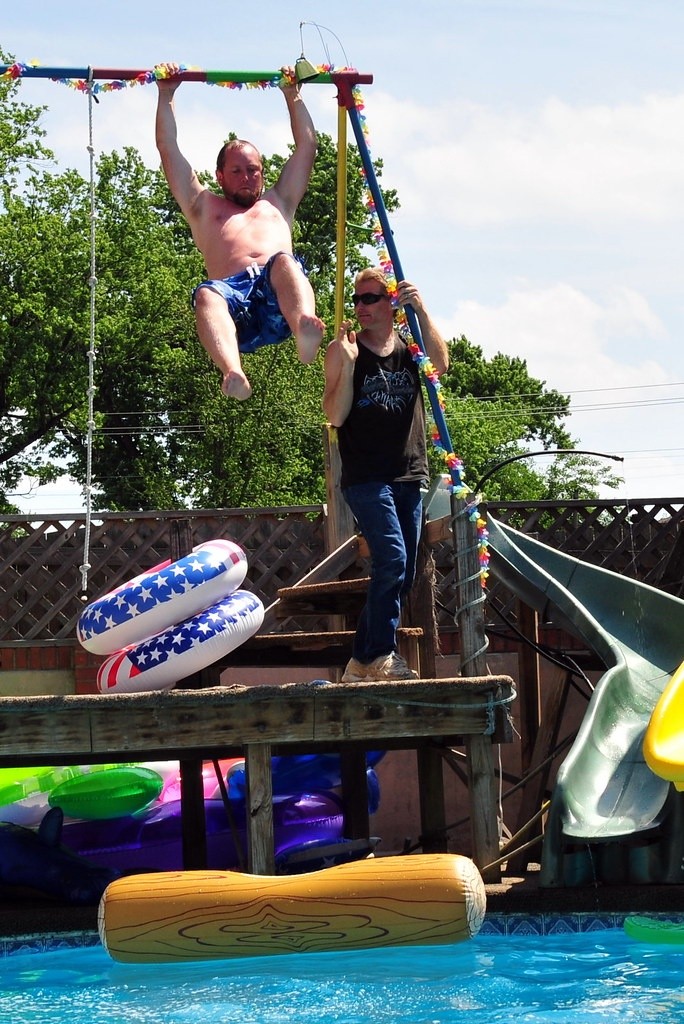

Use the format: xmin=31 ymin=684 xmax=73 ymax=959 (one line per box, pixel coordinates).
xmin=422 ymin=473 xmax=684 ymax=855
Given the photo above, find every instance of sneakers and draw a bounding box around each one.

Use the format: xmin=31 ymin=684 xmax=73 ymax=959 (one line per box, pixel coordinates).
xmin=341 ymin=658 xmax=368 ymax=682
xmin=365 ymin=652 xmax=418 ymax=680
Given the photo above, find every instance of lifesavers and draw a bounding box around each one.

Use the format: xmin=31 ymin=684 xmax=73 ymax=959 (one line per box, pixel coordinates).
xmin=95 ymin=589 xmax=265 ymax=695
xmin=75 ymin=537 xmax=248 ymax=657
xmin=623 ymin=914 xmax=684 ymax=945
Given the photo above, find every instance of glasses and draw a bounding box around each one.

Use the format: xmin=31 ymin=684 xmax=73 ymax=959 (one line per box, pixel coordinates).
xmin=352 ymin=292 xmax=385 ymax=306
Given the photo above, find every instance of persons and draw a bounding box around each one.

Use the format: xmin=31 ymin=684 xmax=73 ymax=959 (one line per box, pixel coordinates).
xmin=156 ymin=62 xmax=324 ymax=402
xmin=322 ymin=268 xmax=449 ymax=682
